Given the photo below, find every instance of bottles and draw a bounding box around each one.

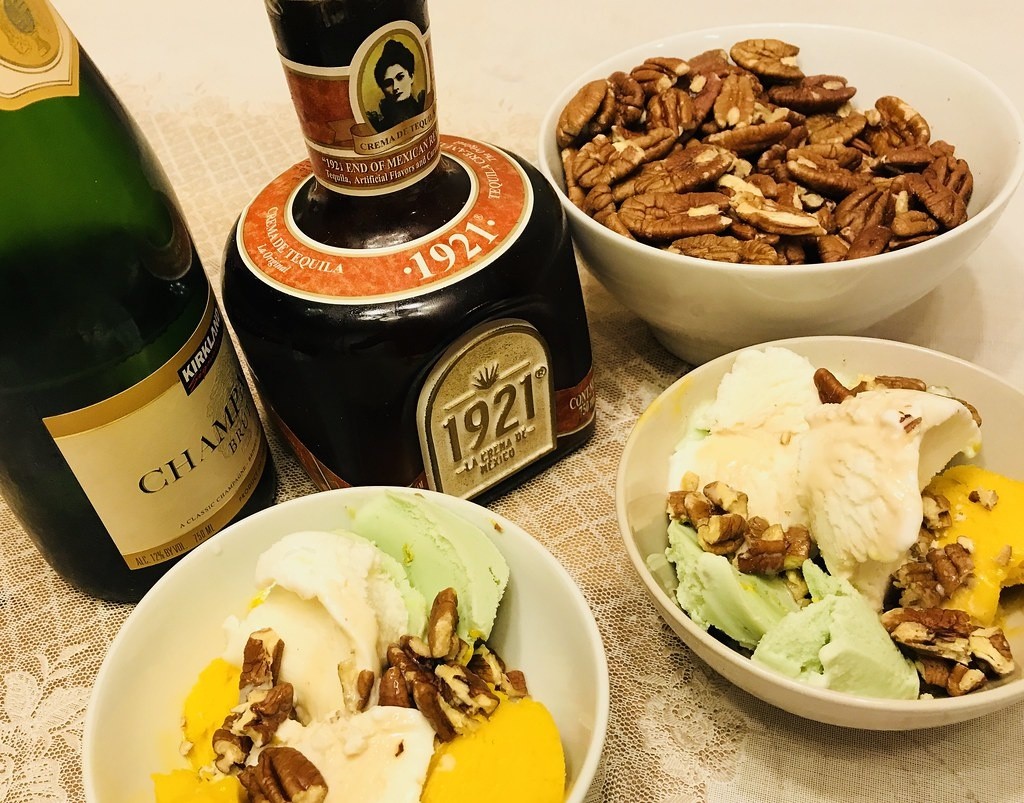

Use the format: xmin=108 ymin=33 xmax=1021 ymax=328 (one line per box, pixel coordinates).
xmin=3 ymin=0 xmax=277 ymax=609
xmin=221 ymin=3 xmax=598 ymax=510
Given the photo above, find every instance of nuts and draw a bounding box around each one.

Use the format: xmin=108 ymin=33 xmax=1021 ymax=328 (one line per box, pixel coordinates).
xmin=671 ymin=368 xmax=1016 ymax=691
xmin=562 ymin=36 xmax=975 ymax=266
xmin=214 ymin=596 xmax=540 ymax=803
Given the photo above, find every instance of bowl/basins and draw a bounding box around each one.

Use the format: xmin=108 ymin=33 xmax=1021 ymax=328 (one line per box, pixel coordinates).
xmin=616 ymin=335 xmax=1023 ymax=732
xmin=541 ymin=21 xmax=1024 ymax=371
xmin=78 ymin=488 xmax=605 ymax=803
